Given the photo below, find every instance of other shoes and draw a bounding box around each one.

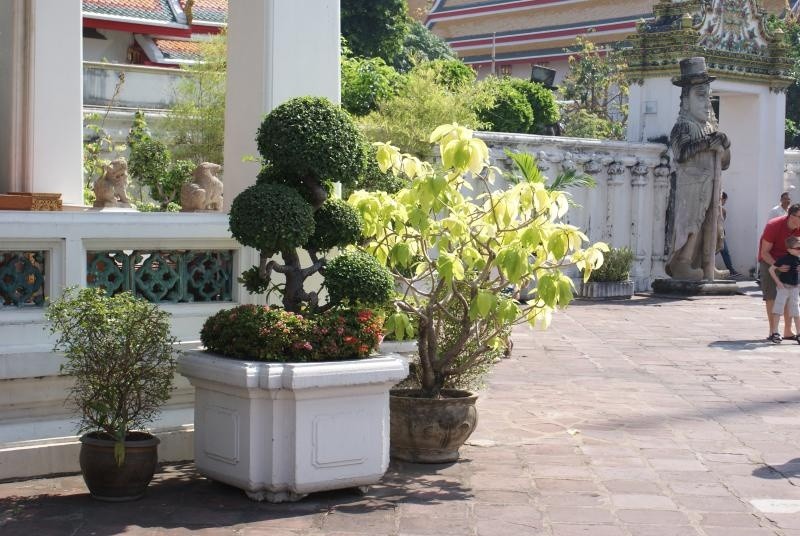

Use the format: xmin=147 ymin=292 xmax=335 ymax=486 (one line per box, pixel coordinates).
xmin=771 ymin=333 xmax=783 ymax=344
xmin=796 ymin=335 xmax=800 ymax=344
xmin=730 ymin=270 xmax=741 ymax=278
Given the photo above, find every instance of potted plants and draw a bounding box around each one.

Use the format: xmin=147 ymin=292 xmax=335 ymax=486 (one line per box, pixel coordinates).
xmin=581 ymin=250 xmax=635 ymax=297
xmin=392 ymin=171 xmax=561 ymax=462
xmin=54 ymin=286 xmax=163 ymax=497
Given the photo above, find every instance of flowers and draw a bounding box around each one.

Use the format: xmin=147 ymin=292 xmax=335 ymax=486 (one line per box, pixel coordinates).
xmin=205 ymin=309 xmax=382 ymax=358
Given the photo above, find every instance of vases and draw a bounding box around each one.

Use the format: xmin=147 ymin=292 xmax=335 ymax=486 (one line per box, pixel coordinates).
xmin=186 ymin=350 xmax=386 ymax=494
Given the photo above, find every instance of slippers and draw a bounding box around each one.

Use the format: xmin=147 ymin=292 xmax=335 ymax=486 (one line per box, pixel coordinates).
xmin=784 ymin=335 xmax=797 ymax=340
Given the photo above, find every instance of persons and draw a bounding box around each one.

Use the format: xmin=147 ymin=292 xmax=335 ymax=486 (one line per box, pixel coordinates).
xmin=766 ymin=192 xmax=791 ymax=223
xmin=769 ymin=236 xmax=800 ymax=345
xmin=758 ymin=203 xmax=800 ymax=344
xmin=715 ymin=192 xmax=745 ymax=280
xmin=665 ymin=57 xmax=731 ymax=281
